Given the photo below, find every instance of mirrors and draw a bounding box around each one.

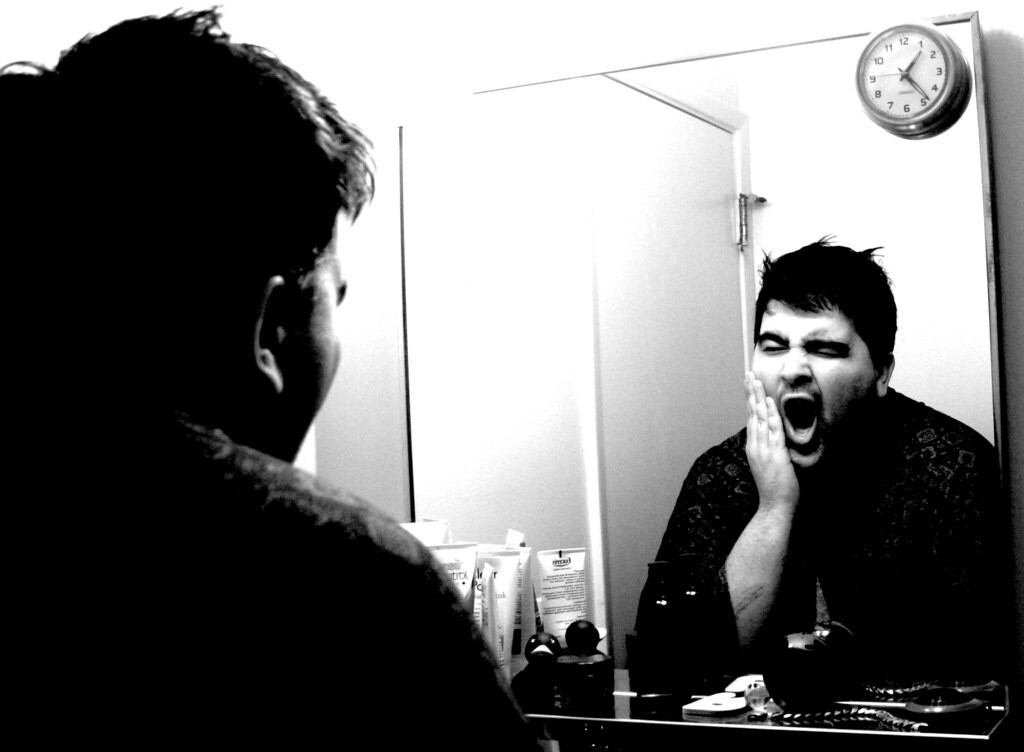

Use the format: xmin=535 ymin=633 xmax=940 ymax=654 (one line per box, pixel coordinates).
xmin=396 ymin=10 xmax=1016 ymax=752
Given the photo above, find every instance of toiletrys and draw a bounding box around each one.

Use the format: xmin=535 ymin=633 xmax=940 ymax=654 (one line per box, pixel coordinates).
xmin=478 ymin=527 xmax=589 ymax=682
xmin=399 ymin=521 xmax=520 ymax=685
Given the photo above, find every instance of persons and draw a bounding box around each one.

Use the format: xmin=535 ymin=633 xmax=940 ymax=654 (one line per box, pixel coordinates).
xmin=0 ymin=5 xmax=540 ymax=752
xmin=633 ymin=233 xmax=1008 ymax=699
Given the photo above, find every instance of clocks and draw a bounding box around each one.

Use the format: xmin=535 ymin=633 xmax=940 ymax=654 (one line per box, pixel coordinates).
xmin=856 ymin=22 xmax=959 ymax=138
